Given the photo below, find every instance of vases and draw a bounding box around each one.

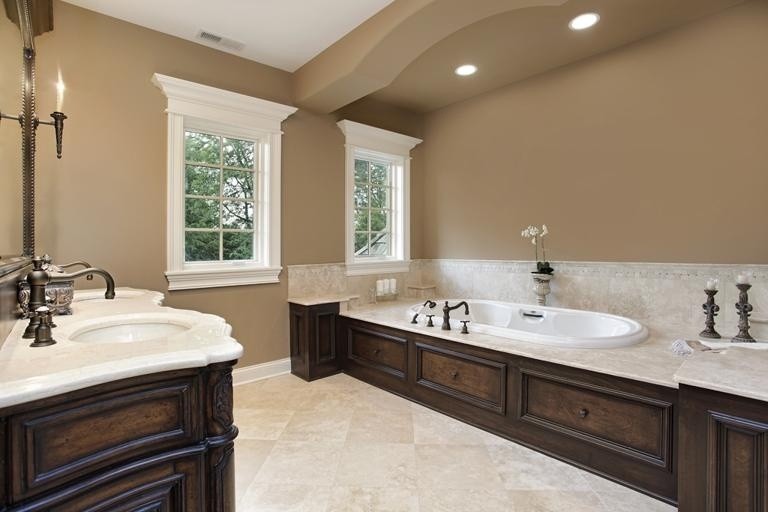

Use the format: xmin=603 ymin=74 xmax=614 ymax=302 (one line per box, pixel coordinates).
xmin=521 ymin=224 xmax=554 ymax=273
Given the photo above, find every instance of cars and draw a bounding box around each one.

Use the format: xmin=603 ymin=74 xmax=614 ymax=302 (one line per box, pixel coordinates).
xmin=375 ymin=279 xmax=396 ymax=295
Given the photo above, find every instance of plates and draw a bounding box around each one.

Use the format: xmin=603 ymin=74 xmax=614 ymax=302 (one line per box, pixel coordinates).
xmin=346 ymin=324 xmax=409 ymax=383
xmin=415 ymin=342 xmax=508 ymax=417
xmin=517 ymin=368 xmax=674 ymax=472
xmin=0 ymin=359 xmax=238 ymax=512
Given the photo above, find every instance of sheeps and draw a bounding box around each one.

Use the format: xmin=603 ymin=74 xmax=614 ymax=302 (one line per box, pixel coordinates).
xmin=442 ymin=300 xmax=469 ymax=330
xmin=22 ymin=256 xmax=115 ymax=338
xmin=58 ymin=261 xmax=93 ymax=280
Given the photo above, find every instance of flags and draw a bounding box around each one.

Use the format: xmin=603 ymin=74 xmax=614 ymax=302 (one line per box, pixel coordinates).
xmin=530 ymin=271 xmax=552 ymax=305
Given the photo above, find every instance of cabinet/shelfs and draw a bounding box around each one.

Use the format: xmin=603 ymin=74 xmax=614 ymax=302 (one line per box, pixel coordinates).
xmin=14 ymin=254 xmax=74 ymax=319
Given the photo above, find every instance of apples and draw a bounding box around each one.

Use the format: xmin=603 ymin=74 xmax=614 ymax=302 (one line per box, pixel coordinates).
xmin=706 ymin=280 xmax=717 ymax=290
xmin=56 ymin=66 xmax=65 ymax=112
xmin=737 ymin=274 xmax=749 ymax=284
